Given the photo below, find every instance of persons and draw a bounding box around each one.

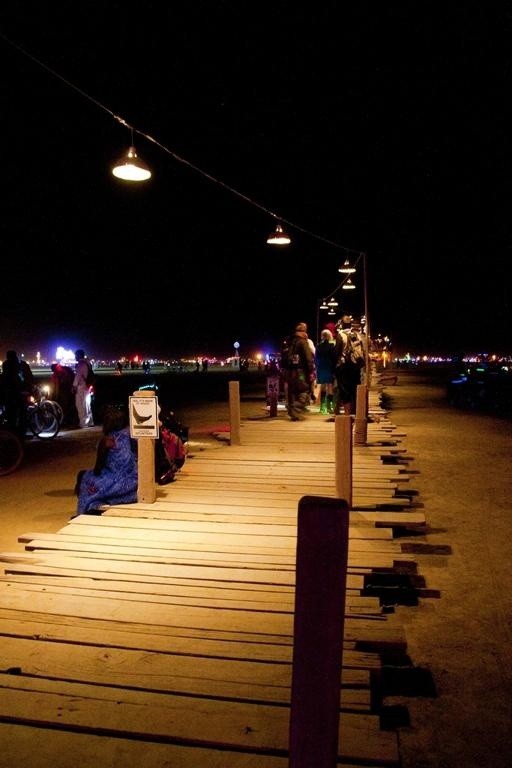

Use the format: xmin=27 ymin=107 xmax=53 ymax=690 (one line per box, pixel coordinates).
xmin=0 ymin=345 xmax=189 ymax=512
xmin=278 ymin=315 xmax=365 ymax=421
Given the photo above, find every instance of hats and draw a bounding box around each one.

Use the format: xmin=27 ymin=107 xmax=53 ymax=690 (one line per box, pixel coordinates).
xmin=296 ymin=322 xmax=307 ymax=330
xmin=340 ymin=316 xmax=351 ymax=324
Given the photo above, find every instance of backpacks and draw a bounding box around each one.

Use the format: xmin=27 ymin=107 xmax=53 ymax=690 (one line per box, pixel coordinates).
xmin=280 ymin=336 xmax=302 ymax=367
xmin=81 ymin=359 xmax=96 ymax=395
xmin=341 ymin=331 xmax=365 ymax=368
xmin=159 ymin=427 xmax=185 ymax=471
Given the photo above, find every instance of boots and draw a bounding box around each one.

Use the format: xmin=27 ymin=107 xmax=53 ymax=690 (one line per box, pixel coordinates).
xmin=320 ymin=392 xmax=327 ymax=413
xmin=327 ymin=395 xmax=334 ymax=414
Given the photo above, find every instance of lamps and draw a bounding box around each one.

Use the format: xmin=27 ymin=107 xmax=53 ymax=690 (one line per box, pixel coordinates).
xmin=338 ymin=248 xmax=357 ymax=274
xmin=342 ymin=274 xmax=356 ymax=290
xmin=266 ymin=217 xmax=292 ymax=245
xmin=319 ymin=293 xmax=339 ymax=316
xmin=112 ymin=127 xmax=152 ymax=182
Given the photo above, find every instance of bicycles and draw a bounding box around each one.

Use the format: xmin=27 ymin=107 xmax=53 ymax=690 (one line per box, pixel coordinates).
xmin=1 ymin=383 xmax=64 ymax=475
